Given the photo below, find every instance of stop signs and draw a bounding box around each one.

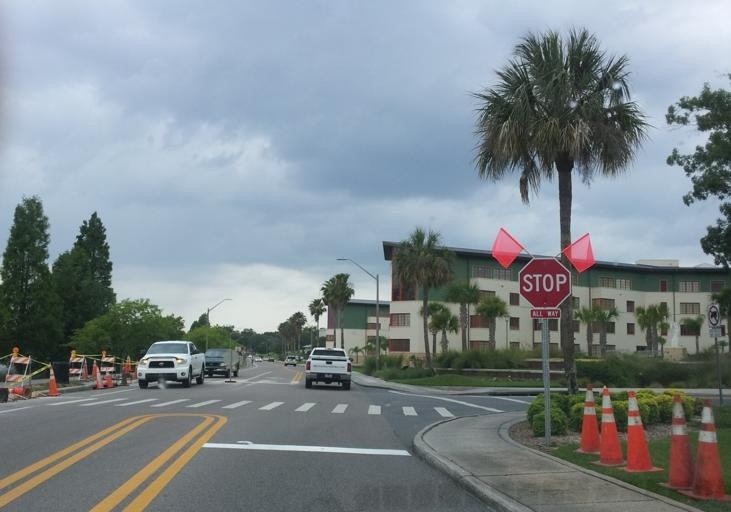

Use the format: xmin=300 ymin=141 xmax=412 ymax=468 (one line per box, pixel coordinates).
xmin=518 ymin=258 xmax=572 ymax=308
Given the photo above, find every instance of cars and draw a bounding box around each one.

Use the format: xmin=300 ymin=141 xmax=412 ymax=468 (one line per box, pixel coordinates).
xmin=285 ymin=356 xmax=297 ymax=367
xmin=306 ymin=348 xmax=354 ymax=389
xmin=248 ymin=355 xmax=275 ymax=362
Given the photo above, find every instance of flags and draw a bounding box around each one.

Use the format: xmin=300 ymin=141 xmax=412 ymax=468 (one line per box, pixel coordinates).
xmin=491 ymin=228 xmax=525 ymax=270
xmin=563 ymin=233 xmax=596 ymax=273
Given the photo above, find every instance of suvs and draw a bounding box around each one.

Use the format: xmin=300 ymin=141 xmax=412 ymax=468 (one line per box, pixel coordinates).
xmin=137 ymin=340 xmax=205 ymax=388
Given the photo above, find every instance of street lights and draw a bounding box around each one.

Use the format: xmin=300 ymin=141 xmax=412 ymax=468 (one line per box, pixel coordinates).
xmin=206 ymin=299 xmax=232 ymax=350
xmin=337 ymin=258 xmax=380 ymax=373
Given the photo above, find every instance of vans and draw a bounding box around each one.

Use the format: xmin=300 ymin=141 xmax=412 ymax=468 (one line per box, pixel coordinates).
xmin=205 ymin=348 xmax=241 ymax=378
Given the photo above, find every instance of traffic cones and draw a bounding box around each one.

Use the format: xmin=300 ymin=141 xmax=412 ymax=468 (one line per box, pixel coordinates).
xmin=92 ymin=359 xmax=115 ymax=389
xmin=47 ymin=367 xmax=61 ymax=396
xmin=574 ymin=383 xmax=665 ymax=473
xmin=8 ymin=386 xmax=32 ymax=399
xmin=124 ymin=355 xmax=134 ymax=375
xmin=657 ymin=392 xmax=731 ymax=501
